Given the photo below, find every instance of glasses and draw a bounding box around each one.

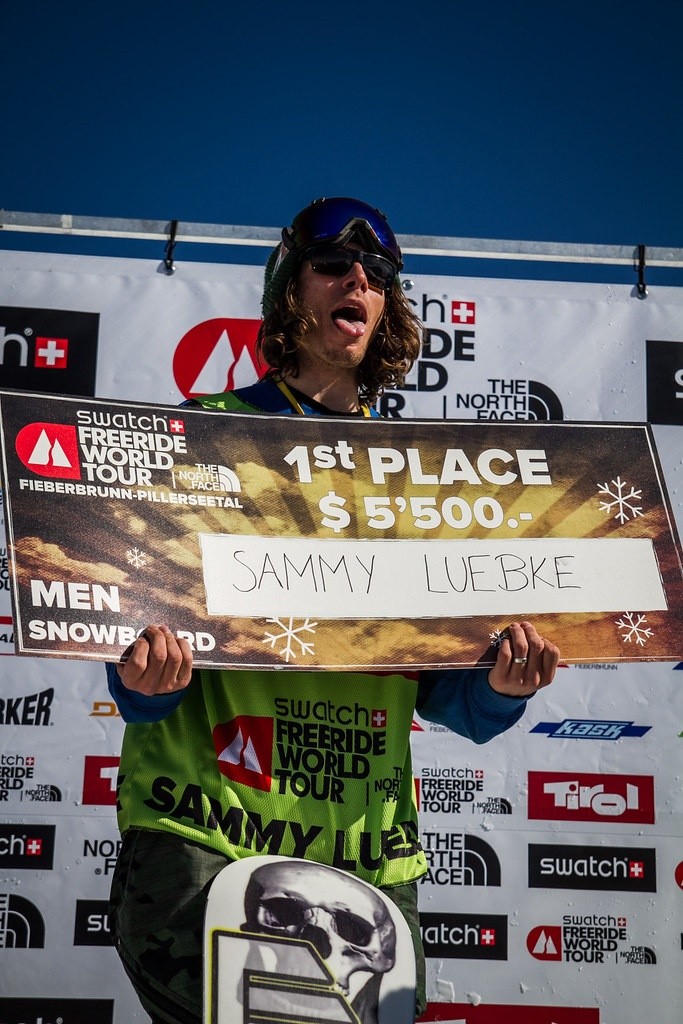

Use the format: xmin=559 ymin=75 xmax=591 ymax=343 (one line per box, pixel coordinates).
xmin=271 ymin=197 xmax=404 ymax=275
xmin=293 ymin=245 xmax=397 ymax=291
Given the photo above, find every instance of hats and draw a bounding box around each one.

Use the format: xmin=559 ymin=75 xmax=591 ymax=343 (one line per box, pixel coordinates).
xmin=262 ymin=241 xmax=401 ymax=318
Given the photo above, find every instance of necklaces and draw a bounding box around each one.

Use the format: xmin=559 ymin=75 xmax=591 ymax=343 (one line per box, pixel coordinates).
xmin=274 ymin=375 xmax=372 ymax=417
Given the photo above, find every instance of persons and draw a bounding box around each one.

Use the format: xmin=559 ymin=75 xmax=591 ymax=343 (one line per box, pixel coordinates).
xmin=106 ymin=197 xmax=560 ymax=1024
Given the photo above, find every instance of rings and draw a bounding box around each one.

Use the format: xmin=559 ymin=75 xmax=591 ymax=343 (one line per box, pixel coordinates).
xmin=513 ymin=658 xmax=528 ymax=663
